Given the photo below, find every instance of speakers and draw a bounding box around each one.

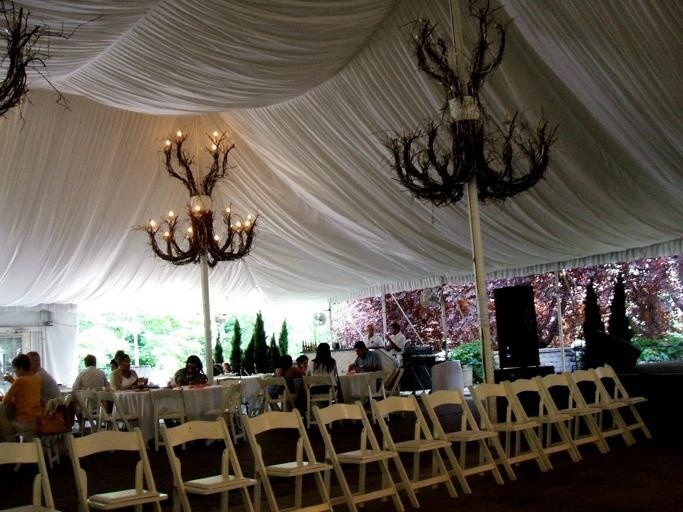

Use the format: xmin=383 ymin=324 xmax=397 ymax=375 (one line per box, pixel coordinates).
xmin=493 ymin=284 xmax=541 ymax=370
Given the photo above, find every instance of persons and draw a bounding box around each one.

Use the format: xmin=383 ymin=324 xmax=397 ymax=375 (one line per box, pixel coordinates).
xmin=221 ymin=361 xmax=232 ymax=374
xmin=306 ymin=342 xmax=340 ymax=430
xmin=110 ymin=351 xmax=123 ymax=371
xmin=266 ymin=354 xmax=304 ymax=411
xmin=110 ymin=350 xmax=149 ymax=431
xmin=71 ymin=353 xmax=111 ymax=436
xmin=361 ymin=324 xmax=384 ymax=348
xmin=383 ymin=322 xmax=406 ymax=391
xmin=294 ymin=354 xmax=309 ymax=374
xmin=348 ymin=341 xmax=383 ymax=409
xmin=165 ymin=353 xmax=207 ymax=386
xmin=0 ymin=353 xmax=43 ymax=474
xmin=26 ymin=350 xmax=62 ymax=416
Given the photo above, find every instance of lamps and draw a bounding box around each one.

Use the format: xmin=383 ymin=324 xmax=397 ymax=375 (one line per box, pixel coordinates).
xmin=385 ymin=0 xmax=560 ymax=210
xmin=135 ymin=120 xmax=263 ymax=266
xmin=1 ymin=0 xmax=103 ymax=132
